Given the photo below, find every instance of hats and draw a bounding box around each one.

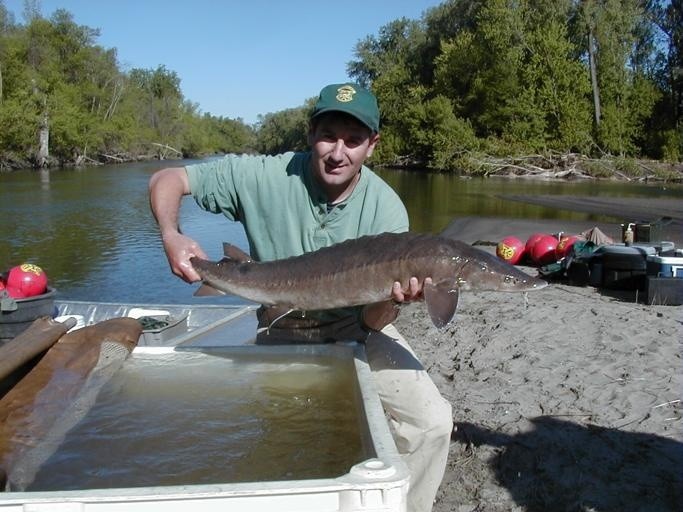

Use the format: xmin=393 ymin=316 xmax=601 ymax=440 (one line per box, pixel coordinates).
xmin=309 ymin=84 xmax=380 ymax=134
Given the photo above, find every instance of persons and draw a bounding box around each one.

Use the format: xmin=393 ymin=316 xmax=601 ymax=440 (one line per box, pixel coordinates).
xmin=148 ymin=81 xmax=453 ymax=512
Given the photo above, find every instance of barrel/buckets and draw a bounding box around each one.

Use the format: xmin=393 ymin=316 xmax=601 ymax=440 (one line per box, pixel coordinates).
xmin=1 ymin=285 xmax=59 ymax=347
xmin=600 ymin=240 xmax=676 ymax=293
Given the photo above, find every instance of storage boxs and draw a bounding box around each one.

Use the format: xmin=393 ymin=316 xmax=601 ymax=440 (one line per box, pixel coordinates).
xmin=646 ymin=253 xmax=683 ymax=278
xmin=136 ymin=314 xmax=188 ymax=345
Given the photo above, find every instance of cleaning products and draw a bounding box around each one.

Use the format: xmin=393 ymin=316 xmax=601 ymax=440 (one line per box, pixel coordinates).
xmin=623 ymin=222 xmax=637 ymax=247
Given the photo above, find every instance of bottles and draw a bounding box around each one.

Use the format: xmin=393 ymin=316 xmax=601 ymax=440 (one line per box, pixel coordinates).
xmin=624 ymin=228 xmax=633 ymax=246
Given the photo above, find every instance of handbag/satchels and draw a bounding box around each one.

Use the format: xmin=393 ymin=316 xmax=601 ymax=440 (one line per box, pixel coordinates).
xmin=538 ymin=240 xmax=603 ymax=285
